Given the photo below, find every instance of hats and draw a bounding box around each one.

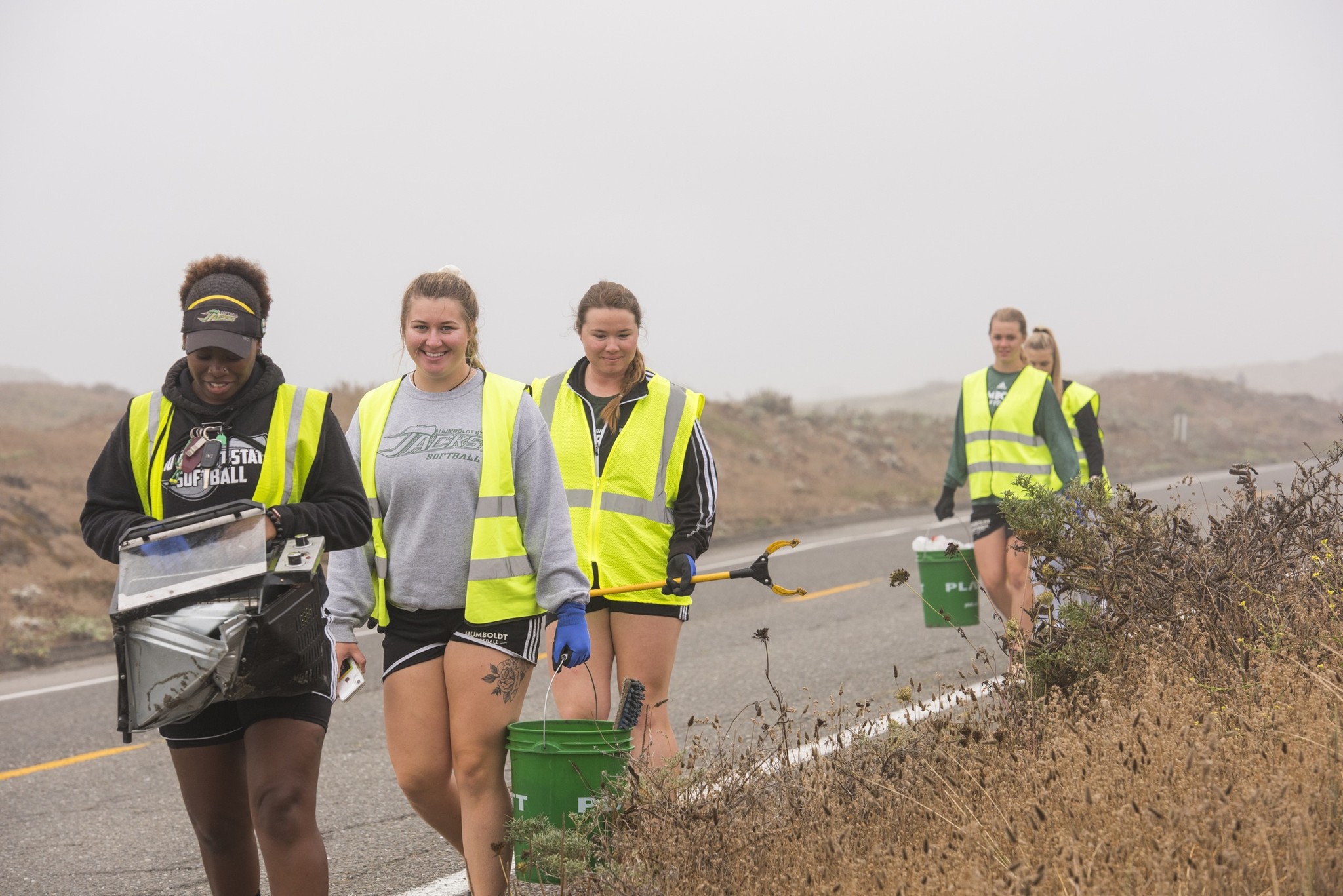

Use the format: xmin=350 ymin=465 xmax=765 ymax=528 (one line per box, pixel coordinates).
xmin=181 ymin=274 xmax=268 ymax=359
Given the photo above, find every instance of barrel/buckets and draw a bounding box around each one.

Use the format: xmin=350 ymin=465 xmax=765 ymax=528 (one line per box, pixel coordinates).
xmin=914 ymin=509 xmax=978 ymax=628
xmin=504 ymin=654 xmax=636 ymax=885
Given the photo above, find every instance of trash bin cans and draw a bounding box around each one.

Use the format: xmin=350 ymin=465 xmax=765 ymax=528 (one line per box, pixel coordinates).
xmin=505 ymin=654 xmax=634 ymax=885
xmin=917 ymin=515 xmax=979 ymax=628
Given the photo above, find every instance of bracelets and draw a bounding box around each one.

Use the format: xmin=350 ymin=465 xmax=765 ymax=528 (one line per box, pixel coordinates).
xmin=263 ymin=508 xmax=284 ymax=552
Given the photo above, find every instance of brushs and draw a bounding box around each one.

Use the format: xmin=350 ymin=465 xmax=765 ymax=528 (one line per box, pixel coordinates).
xmin=612 ymin=677 xmax=646 ymax=731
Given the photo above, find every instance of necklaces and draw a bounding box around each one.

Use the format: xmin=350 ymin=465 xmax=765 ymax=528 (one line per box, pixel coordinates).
xmin=413 ymin=362 xmax=471 ymax=392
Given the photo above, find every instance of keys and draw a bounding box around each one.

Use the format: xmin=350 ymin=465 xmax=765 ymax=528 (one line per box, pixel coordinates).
xmin=200 ymin=440 xmax=222 ymax=489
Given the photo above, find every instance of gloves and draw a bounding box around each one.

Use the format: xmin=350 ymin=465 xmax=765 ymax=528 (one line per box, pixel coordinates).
xmin=935 ymin=485 xmax=956 ymax=521
xmin=135 ymin=532 xmax=191 ymax=571
xmin=661 ymin=554 xmax=697 ymax=596
xmin=551 ymin=603 xmax=592 ymax=673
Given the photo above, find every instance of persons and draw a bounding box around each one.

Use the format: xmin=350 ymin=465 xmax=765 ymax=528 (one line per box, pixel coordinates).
xmin=933 ymin=307 xmax=1083 ymax=675
xmin=80 ymin=257 xmax=375 ymax=895
xmin=324 ymin=272 xmax=592 ymax=896
xmin=532 ymin=282 xmax=718 ymax=806
xmin=1025 ymin=326 xmax=1110 ymax=537
